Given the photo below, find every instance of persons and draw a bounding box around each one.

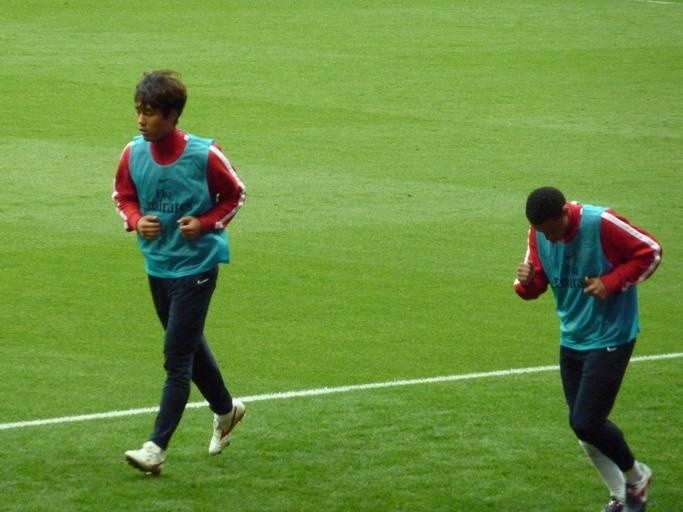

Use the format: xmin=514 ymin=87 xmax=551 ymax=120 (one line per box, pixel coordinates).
xmin=511 ymin=186 xmax=663 ymax=512
xmin=111 ymin=67 xmax=247 ymax=476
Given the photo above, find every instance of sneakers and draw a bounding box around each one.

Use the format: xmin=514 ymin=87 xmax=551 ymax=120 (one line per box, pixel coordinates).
xmin=602 ymin=495 xmax=626 ymax=512
xmin=625 ymin=462 xmax=652 ymax=512
xmin=209 ymin=399 xmax=246 ymax=455
xmin=125 ymin=442 xmax=166 ymax=474
